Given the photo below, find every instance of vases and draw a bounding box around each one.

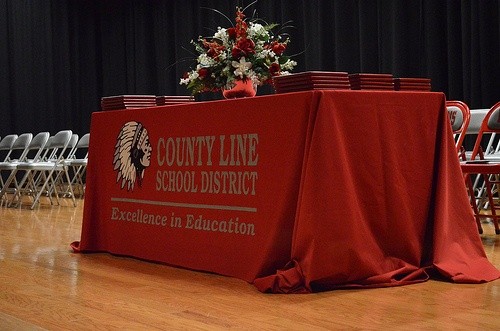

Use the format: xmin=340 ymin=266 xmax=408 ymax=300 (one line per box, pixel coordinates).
xmin=223 ymin=78 xmax=257 ymax=100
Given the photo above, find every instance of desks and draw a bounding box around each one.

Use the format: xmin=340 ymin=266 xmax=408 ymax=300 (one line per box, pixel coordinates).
xmin=70 ymin=90 xmax=500 ymax=293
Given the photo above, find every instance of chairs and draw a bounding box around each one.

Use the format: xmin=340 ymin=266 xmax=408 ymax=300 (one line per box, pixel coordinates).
xmin=446 ymin=101 xmax=500 ymax=234
xmin=0 ymin=130 xmax=90 ymax=209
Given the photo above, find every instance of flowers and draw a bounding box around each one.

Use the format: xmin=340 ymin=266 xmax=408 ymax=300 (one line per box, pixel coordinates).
xmin=167 ymin=0 xmax=303 ymax=102
